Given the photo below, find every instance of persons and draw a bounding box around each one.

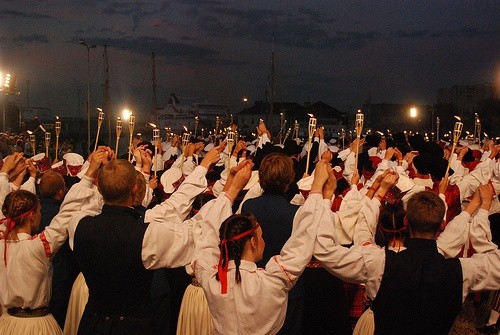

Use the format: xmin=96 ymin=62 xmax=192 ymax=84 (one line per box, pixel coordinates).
xmin=0 ymin=121 xmax=500 ymax=335
